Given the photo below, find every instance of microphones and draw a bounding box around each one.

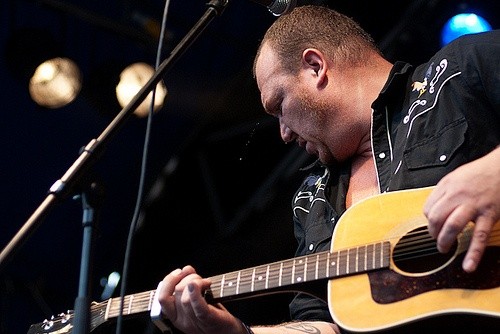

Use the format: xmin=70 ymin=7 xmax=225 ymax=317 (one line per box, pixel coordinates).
xmin=253 ymin=0 xmax=296 ymax=17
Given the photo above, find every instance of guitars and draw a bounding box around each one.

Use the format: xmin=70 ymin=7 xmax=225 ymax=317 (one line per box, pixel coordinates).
xmin=27 ymin=179 xmax=499 ymax=334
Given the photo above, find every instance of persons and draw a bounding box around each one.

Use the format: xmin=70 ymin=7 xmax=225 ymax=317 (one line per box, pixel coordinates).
xmin=157 ymin=4 xmax=500 ymax=334
